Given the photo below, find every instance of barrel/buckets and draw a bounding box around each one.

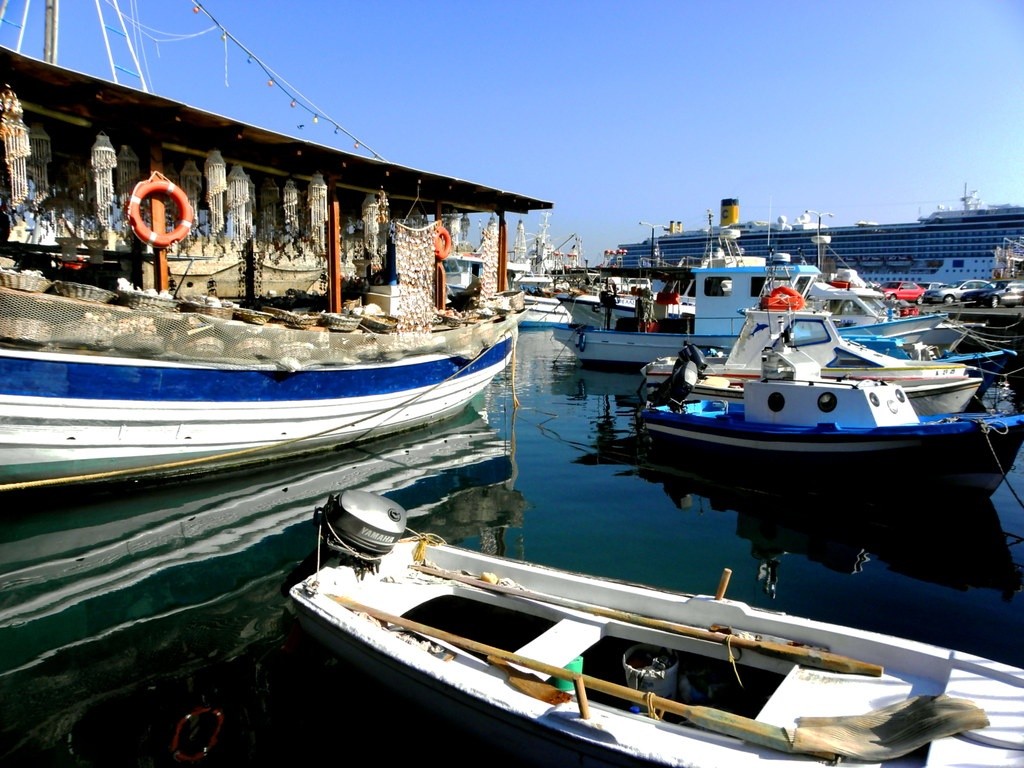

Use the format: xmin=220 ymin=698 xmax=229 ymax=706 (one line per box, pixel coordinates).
xmin=622 ymin=642 xmax=680 ymax=713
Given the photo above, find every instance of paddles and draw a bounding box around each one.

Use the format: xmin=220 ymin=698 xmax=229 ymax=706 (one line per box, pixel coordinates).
xmin=408 ymin=562 xmax=886 ymax=678
xmin=322 ymin=592 xmax=838 ymax=759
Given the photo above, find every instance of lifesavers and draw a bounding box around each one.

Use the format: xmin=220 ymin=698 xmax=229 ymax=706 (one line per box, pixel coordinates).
xmin=431 ymin=226 xmax=451 ymax=259
xmin=128 ymin=181 xmax=195 ymax=246
xmin=770 ymin=286 xmax=804 ymax=310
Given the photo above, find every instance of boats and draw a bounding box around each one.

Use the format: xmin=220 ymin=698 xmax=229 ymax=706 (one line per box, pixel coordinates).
xmin=290 ymin=488 xmax=1024 ymax=768
xmin=1 ymin=42 xmax=560 ymax=483
xmin=393 ymin=183 xmax=1024 ymax=454
